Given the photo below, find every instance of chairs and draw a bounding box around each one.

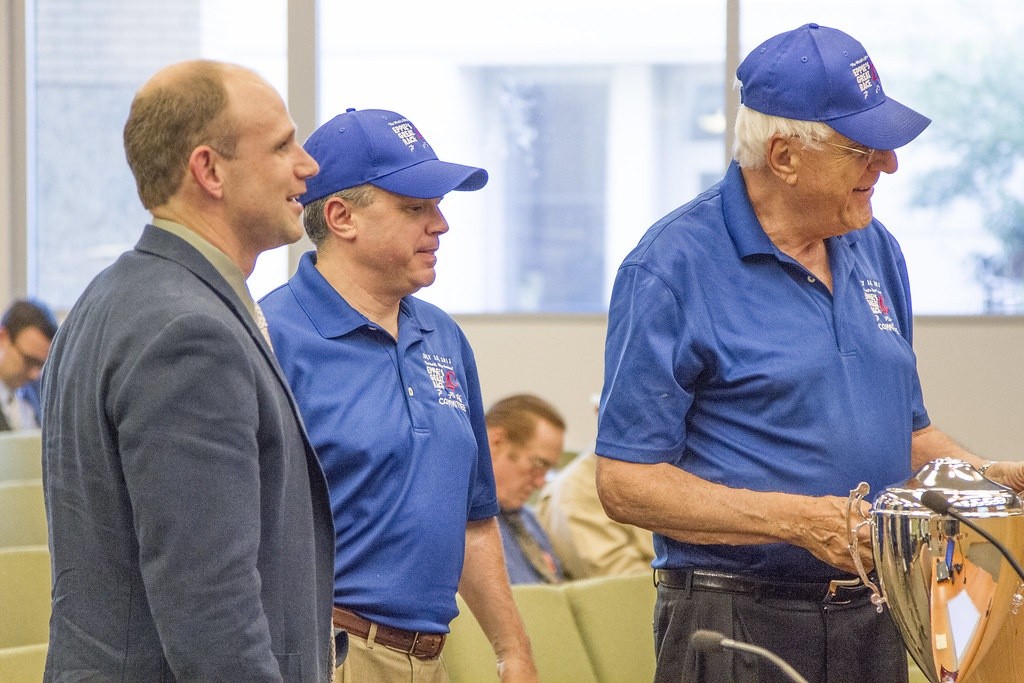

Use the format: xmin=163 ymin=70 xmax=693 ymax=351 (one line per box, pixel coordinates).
xmin=0 ymin=428 xmax=57 ymax=682
xmin=440 ymin=563 xmax=659 ymax=683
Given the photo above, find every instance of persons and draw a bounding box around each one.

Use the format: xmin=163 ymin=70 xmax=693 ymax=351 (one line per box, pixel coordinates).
xmin=0 ymin=297 xmax=60 ymax=434
xmin=474 ymin=397 xmax=573 ymax=591
xmin=255 ymin=105 xmax=544 ymax=683
xmin=590 ymin=20 xmax=1021 ymax=683
xmin=36 ymin=59 xmax=339 ymax=682
xmin=535 ymin=393 xmax=662 ymax=584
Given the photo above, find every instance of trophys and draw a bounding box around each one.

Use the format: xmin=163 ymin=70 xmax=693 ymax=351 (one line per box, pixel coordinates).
xmin=842 ymin=456 xmax=1024 ymax=683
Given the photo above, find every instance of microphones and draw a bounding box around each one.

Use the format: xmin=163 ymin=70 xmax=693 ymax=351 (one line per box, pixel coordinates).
xmin=921 ymin=491 xmax=1024 ymax=582
xmin=691 ymin=631 xmax=809 ymax=683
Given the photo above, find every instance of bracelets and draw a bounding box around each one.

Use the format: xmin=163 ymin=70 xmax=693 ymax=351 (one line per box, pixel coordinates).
xmin=976 ymin=460 xmax=1000 ymax=478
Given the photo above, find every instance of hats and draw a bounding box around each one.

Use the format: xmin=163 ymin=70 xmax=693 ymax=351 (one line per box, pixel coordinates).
xmin=294 ymin=108 xmax=490 ymax=208
xmin=735 ymin=22 xmax=932 ymax=152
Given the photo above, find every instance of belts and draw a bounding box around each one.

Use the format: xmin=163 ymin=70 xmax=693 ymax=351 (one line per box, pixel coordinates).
xmin=656 ymin=567 xmax=877 ymax=606
xmin=334 ymin=605 xmax=447 ymax=661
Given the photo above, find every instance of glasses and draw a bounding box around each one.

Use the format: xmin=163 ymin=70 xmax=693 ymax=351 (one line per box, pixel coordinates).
xmin=523 ymin=445 xmax=560 ymax=483
xmin=13 ymin=341 xmax=45 ymax=369
xmin=787 ymin=129 xmax=875 ymax=162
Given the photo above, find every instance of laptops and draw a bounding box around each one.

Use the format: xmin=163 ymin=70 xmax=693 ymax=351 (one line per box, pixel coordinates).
xmin=936 ymin=536 xmax=955 ymax=583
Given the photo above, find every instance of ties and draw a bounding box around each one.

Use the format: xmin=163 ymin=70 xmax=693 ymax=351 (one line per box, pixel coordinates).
xmin=503 ymin=510 xmax=562 ymax=585
xmin=9 ymin=392 xmax=23 ymax=431
